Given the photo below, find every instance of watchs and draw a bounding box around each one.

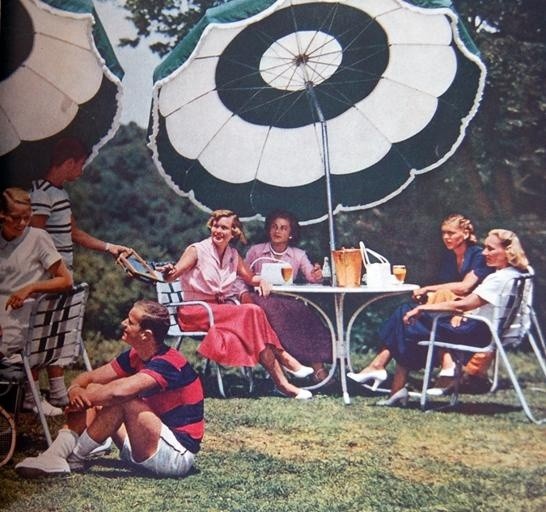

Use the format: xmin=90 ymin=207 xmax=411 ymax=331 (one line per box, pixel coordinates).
xmin=105 ymin=242 xmax=111 ymax=253
xmin=66 ymin=384 xmax=81 ymax=398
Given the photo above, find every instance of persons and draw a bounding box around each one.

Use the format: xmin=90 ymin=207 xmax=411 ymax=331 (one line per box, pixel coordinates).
xmin=153 ymin=209 xmax=314 ymax=401
xmin=15 ymin=300 xmax=204 ymax=479
xmin=0 ymin=186 xmax=74 ymax=367
xmin=235 ymin=210 xmax=339 ymax=388
xmin=346 ymin=229 xmax=534 ymax=407
xmin=410 ymin=213 xmax=495 ymax=397
xmin=19 ymin=134 xmax=133 ymax=416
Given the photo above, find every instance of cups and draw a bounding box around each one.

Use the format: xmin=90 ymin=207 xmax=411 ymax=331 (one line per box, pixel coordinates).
xmin=281 ymin=266 xmax=293 ymax=288
xmin=392 ymin=265 xmax=407 ymax=285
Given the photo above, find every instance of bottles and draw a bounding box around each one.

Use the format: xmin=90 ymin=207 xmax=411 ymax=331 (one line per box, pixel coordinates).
xmin=322 ymin=256 xmax=330 ymax=285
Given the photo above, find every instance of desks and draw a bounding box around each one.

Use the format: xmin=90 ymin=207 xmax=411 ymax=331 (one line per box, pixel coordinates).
xmin=268 ymin=283 xmax=422 ymax=403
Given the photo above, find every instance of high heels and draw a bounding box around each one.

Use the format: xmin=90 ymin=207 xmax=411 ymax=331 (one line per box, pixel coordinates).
xmin=427 ymin=386 xmax=446 ymax=397
xmin=346 ymin=369 xmax=388 ymax=390
xmin=439 ymin=360 xmax=458 ymax=378
xmin=275 ymin=383 xmax=312 ymax=399
xmin=282 ymin=357 xmax=314 ymax=379
xmin=375 ymin=388 xmax=410 ymax=407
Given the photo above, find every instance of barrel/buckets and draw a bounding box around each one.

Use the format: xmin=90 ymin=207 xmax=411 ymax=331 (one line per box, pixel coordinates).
xmin=334 ymin=247 xmax=362 ymax=288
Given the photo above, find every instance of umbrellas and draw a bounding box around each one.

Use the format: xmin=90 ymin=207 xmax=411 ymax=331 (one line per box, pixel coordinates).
xmin=0 ymin=0 xmax=125 ymax=205
xmin=145 ymin=0 xmax=488 ymax=287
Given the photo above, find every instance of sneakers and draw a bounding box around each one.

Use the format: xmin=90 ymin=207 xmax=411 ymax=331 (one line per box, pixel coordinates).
xmin=67 ymin=450 xmax=98 ymax=470
xmin=21 ymin=395 xmax=64 ymax=417
xmin=15 ymin=454 xmax=71 ymax=477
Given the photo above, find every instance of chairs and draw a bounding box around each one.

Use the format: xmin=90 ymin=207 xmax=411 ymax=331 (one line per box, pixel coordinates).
xmin=154 ymin=261 xmax=254 ymax=396
xmin=503 ymin=267 xmax=545 ymax=378
xmin=0 ymin=281 xmax=93 ymax=449
xmin=421 ymin=274 xmax=545 ymax=428
